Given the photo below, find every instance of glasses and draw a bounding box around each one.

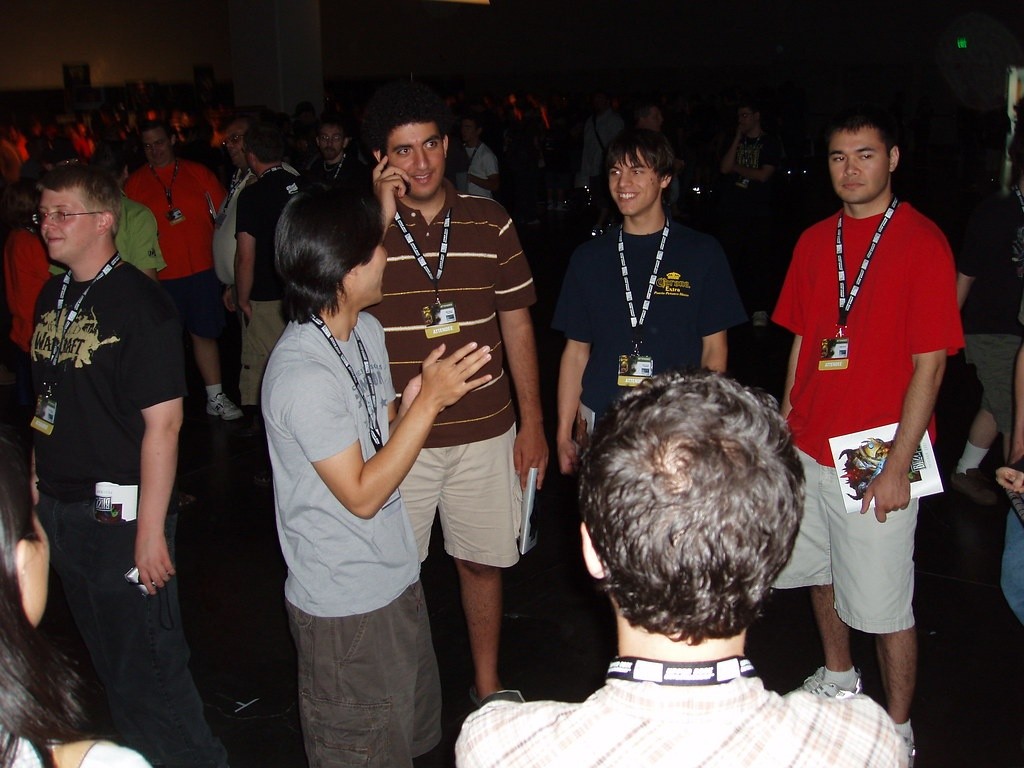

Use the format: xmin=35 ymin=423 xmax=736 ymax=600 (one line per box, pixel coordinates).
xmin=32 ymin=209 xmax=104 ymax=225
xmin=222 ymin=134 xmax=244 ymax=147
xmin=142 ymin=136 xmax=170 ymax=151
xmin=319 ymin=134 xmax=342 ymax=143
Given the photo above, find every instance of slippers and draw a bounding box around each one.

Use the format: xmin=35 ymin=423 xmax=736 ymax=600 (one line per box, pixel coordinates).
xmin=466 ymin=686 xmax=525 ymax=711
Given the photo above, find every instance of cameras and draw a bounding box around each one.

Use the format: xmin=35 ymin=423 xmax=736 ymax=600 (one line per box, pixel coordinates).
xmin=123 ymin=564 xmax=157 ymax=595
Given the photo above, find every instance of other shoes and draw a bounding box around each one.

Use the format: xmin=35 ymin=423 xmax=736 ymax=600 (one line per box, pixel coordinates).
xmin=233 ymin=417 xmax=265 ymax=436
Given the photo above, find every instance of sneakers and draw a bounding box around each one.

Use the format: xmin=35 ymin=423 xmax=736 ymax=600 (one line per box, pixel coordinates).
xmin=783 ymin=667 xmax=863 ymax=699
xmin=951 ymin=466 xmax=998 ymax=506
xmin=896 ymin=726 xmax=916 ymax=768
xmin=206 ymin=392 xmax=245 ymax=420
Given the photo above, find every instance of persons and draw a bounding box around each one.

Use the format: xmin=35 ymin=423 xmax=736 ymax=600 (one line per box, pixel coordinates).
xmin=454 ymin=369 xmax=909 ymax=768
xmin=721 ymin=102 xmax=778 ymax=326
xmin=449 ymin=92 xmax=625 ymax=228
xmin=259 ymin=194 xmax=492 ymax=768
xmin=906 ymin=96 xmax=1024 ymax=624
xmin=634 ymin=103 xmax=721 ymax=225
xmin=0 ymin=439 xmax=153 ymax=768
xmin=17 ymin=163 xmax=230 ymax=768
xmin=552 ymin=130 xmax=749 ymax=477
xmin=0 ymin=63 xmax=373 ymax=420
xmin=363 ymin=103 xmax=549 ymax=706
xmin=774 ymin=111 xmax=966 ymax=768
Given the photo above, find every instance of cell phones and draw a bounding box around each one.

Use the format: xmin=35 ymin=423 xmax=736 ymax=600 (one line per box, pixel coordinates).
xmin=381 ymin=162 xmax=411 ymax=196
